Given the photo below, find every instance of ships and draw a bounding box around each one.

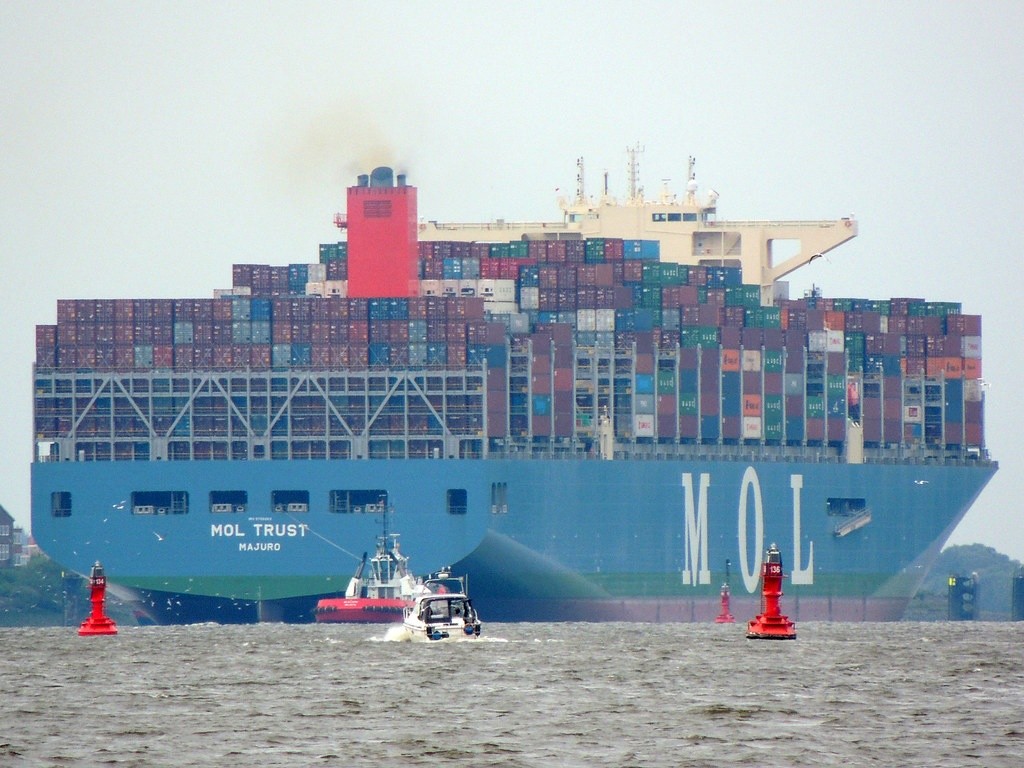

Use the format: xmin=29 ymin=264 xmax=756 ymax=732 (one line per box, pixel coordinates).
xmin=30 ymin=151 xmax=1001 ymax=625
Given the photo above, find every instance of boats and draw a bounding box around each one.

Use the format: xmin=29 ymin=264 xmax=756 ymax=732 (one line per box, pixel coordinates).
xmin=310 ymin=501 xmax=431 ymax=623
xmin=402 ymin=572 xmax=482 ymax=645
